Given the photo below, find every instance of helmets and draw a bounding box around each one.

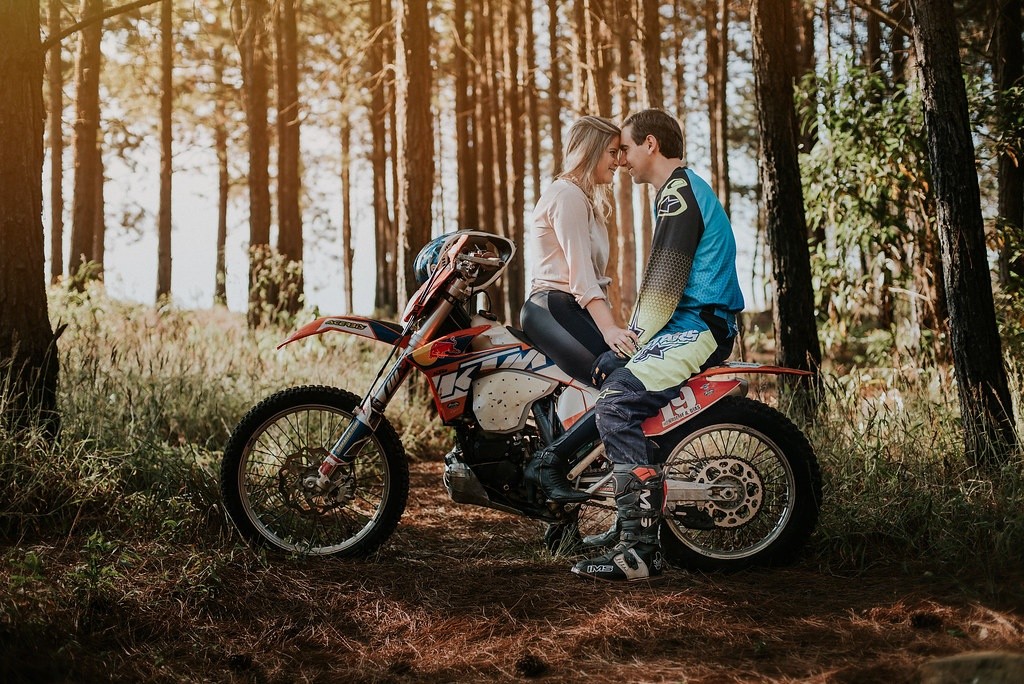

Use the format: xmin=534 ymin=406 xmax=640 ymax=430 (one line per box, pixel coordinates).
xmin=413 ymin=229 xmax=516 ymax=297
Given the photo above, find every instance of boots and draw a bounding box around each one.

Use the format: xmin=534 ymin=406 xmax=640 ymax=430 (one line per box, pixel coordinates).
xmin=571 ymin=464 xmax=669 ymax=585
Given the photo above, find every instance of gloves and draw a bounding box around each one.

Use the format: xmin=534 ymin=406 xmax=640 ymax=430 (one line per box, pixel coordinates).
xmin=590 ymin=350 xmax=628 ymax=390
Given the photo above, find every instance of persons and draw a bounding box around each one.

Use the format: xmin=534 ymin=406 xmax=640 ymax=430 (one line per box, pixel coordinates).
xmin=520 ymin=116 xmax=640 ymax=501
xmin=571 ymin=108 xmax=745 ymax=584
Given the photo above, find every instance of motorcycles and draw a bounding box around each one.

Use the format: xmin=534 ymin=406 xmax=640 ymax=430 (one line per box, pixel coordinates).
xmin=220 ymin=228 xmax=824 ymax=575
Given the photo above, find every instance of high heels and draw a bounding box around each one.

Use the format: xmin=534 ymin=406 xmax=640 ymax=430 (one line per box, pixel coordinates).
xmin=521 ymin=450 xmax=589 ymax=505
